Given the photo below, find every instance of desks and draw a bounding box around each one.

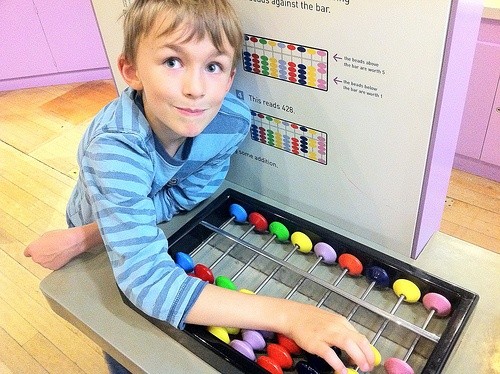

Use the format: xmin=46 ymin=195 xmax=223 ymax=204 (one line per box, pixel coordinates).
xmin=41 ymin=177 xmax=498 ymax=374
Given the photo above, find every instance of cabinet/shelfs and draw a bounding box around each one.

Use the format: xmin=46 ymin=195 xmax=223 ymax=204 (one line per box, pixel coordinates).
xmin=0 ymin=0 xmax=114 ymax=93
xmin=452 ymin=6 xmax=500 ymax=184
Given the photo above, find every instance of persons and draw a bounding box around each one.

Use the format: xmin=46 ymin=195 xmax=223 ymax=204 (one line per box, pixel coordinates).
xmin=24 ymin=0 xmax=375 ymax=374
